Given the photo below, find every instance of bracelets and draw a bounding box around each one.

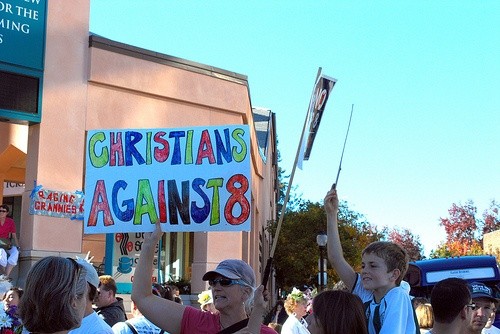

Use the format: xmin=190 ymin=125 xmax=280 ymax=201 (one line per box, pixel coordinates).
xmin=17 ymin=247 xmax=20 ymax=249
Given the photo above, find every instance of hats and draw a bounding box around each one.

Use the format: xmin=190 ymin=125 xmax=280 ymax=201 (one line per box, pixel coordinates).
xmin=202 ymin=259 xmax=255 ymax=283
xmin=75 ymin=258 xmax=100 ymax=291
xmin=468 ymin=282 xmax=498 ymax=303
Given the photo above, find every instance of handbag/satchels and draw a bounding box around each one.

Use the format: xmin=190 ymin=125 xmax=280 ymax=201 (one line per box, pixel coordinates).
xmin=0 ymin=238 xmax=13 ymax=250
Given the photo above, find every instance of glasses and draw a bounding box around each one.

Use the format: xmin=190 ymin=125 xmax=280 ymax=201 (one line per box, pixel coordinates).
xmin=67 ymin=257 xmax=81 ymax=281
xmin=466 ymin=302 xmax=476 ymax=310
xmin=208 ymin=278 xmax=245 ymax=287
xmin=0 ymin=210 xmax=6 ymax=213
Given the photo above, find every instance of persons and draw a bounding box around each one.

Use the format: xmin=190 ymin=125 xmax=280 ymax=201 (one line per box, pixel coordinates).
xmin=425 ymin=278 xmax=474 ymax=334
xmin=132 ymin=218 xmax=278 ymax=334
xmin=247 ymin=286 xmax=369 ymax=334
xmin=92 ymin=275 xmax=125 ymax=327
xmin=112 ymin=283 xmax=184 ymax=334
xmin=0 ymin=204 xmax=21 ymax=282
xmin=280 ymin=291 xmax=312 ymax=334
xmin=19 ymin=256 xmax=87 ymax=334
xmin=67 ymin=258 xmax=114 ymax=334
xmin=3 ymin=287 xmax=30 ymax=334
xmin=467 ymin=282 xmax=500 ymax=334
xmin=413 ymin=297 xmax=435 ymax=334
xmin=324 ymin=184 xmax=419 ymax=334
